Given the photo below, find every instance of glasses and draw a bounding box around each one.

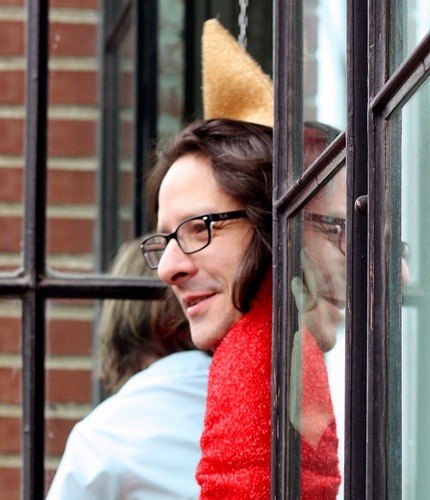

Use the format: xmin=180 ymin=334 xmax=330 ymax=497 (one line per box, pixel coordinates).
xmin=301 ymin=210 xmax=347 ymax=257
xmin=140 ymin=210 xmax=251 ymax=270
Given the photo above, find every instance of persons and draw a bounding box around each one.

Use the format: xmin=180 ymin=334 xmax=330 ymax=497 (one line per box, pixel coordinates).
xmin=402 ymin=242 xmax=410 ymax=288
xmin=140 ymin=118 xmax=272 ymax=500
xmin=44 ymin=236 xmax=213 ymax=500
xmin=291 ymin=245 xmax=346 ymax=472
xmin=300 ymin=120 xmax=347 ymax=500
